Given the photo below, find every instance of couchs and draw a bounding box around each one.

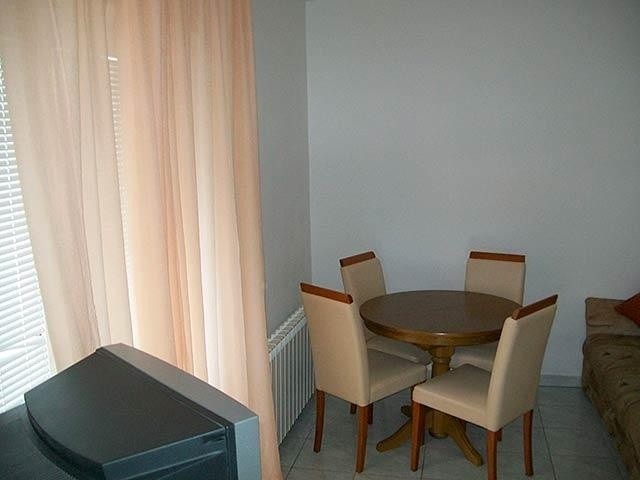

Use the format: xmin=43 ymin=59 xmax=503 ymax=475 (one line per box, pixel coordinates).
xmin=579 ymin=293 xmax=638 ymax=479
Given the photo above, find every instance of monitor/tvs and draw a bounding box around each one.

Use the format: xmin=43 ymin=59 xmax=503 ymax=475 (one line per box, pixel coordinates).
xmin=0 ymin=341 xmax=262 ymax=480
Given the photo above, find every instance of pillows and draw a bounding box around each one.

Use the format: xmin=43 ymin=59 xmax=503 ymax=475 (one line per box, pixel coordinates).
xmin=616 ymin=290 xmax=640 ymax=328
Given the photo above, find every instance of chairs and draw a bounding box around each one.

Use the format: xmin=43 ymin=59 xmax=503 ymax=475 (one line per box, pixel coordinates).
xmin=451 ymin=250 xmax=527 ymax=442
xmin=336 ymin=248 xmax=432 ymax=414
xmin=410 ymin=292 xmax=563 ymax=480
xmin=298 ymin=279 xmax=425 ymax=473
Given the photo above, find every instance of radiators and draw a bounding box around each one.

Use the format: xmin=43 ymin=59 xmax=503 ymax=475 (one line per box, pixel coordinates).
xmin=268 ymin=305 xmax=315 ymax=451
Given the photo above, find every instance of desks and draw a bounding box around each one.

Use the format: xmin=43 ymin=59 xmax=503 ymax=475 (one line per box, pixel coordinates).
xmin=359 ymin=289 xmax=523 ymax=465
